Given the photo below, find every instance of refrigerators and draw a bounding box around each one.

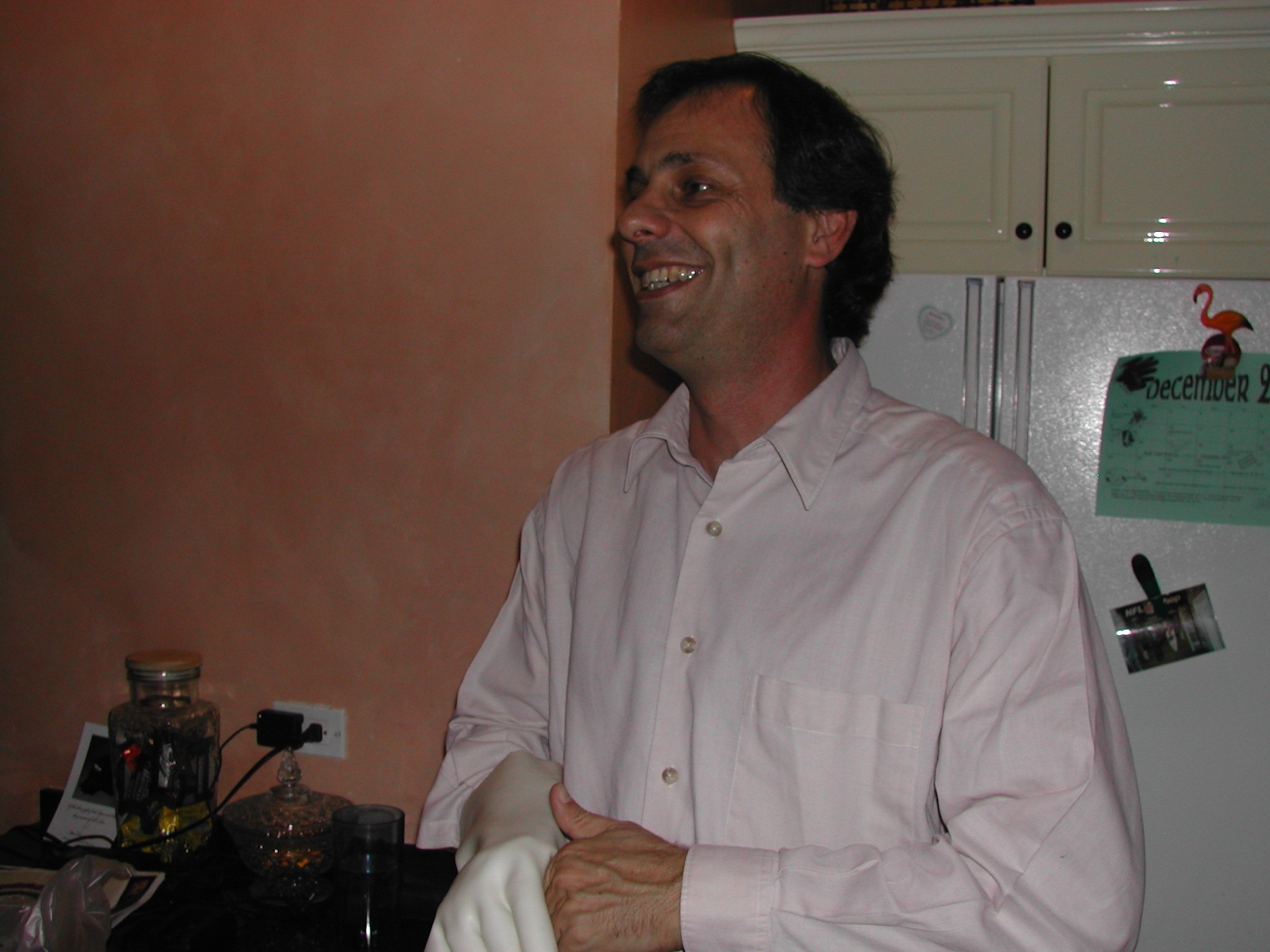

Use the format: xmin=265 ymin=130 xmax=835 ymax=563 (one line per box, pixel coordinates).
xmin=856 ymin=272 xmax=1269 ymax=952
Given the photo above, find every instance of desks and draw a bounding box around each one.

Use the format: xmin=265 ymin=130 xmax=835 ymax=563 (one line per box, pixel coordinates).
xmin=0 ymin=840 xmax=457 ymax=952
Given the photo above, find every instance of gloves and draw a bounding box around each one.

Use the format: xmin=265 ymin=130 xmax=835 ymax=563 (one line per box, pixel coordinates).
xmin=425 ymin=751 xmax=567 ymax=952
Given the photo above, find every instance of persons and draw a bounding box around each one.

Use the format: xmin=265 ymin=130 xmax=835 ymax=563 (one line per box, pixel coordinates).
xmin=418 ymin=51 xmax=1144 ymax=952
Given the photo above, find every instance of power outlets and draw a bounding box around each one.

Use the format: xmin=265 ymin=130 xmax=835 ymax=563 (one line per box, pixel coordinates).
xmin=274 ymin=703 xmax=345 ymax=757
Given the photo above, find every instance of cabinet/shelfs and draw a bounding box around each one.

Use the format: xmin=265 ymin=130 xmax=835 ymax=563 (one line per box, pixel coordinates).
xmin=734 ymin=0 xmax=1270 ymax=276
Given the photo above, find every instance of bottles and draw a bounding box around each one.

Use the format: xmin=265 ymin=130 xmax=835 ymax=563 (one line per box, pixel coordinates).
xmin=221 ymin=744 xmax=355 ymax=904
xmin=104 ymin=649 xmax=218 ymax=874
